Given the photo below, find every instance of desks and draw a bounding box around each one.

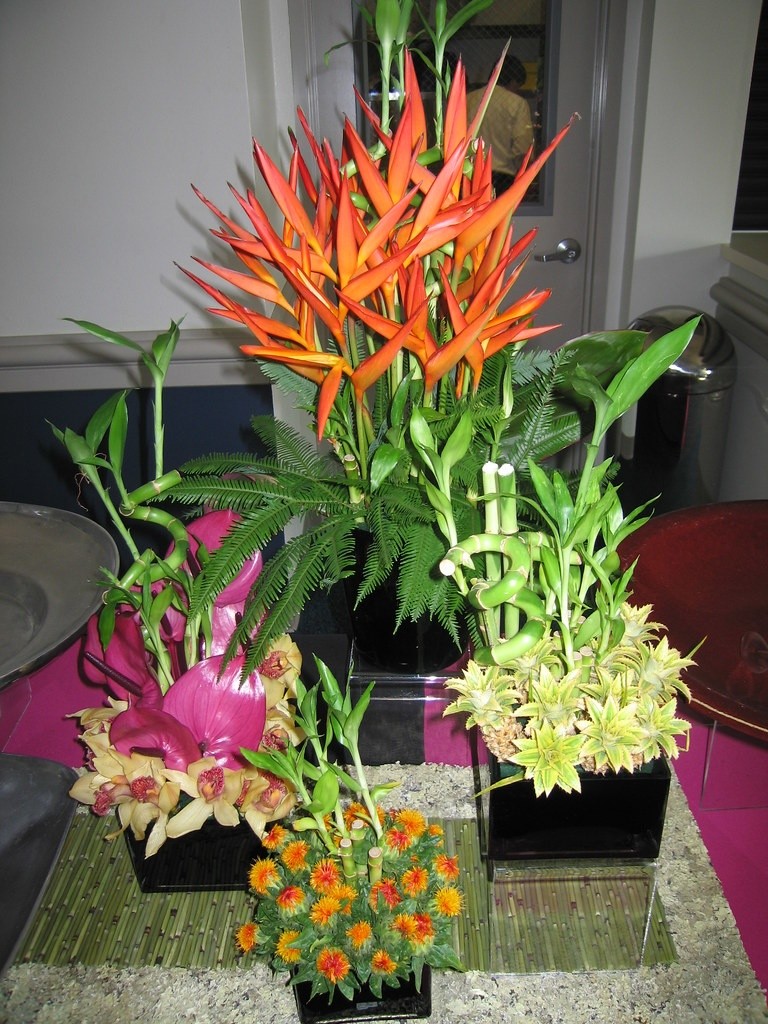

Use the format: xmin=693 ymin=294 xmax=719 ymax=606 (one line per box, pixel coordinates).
xmin=0 ymin=609 xmax=766 ymax=1022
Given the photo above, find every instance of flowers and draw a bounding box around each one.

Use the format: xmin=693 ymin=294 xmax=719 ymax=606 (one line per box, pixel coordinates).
xmin=235 ymin=803 xmax=464 ymax=1004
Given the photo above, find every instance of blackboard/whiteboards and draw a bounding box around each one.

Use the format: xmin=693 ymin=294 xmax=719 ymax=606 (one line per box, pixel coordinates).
xmin=0 ymin=0 xmax=316 ymax=394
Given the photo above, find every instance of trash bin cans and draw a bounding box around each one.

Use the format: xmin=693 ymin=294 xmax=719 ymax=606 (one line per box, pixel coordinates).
xmin=616 ymin=306 xmax=739 ymax=518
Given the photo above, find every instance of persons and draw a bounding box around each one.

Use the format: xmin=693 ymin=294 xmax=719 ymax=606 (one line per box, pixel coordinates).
xmin=367 ymin=41 xmax=383 ymax=94
xmin=464 ymin=53 xmax=536 ymax=200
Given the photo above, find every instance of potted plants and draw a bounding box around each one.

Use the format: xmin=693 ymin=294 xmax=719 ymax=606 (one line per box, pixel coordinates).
xmin=237 ymin=654 xmax=435 ymax=1023
xmin=168 ymin=2 xmax=577 ymax=678
xmin=45 ymin=315 xmax=297 ymax=895
xmin=404 ymin=314 xmax=709 ymax=873
